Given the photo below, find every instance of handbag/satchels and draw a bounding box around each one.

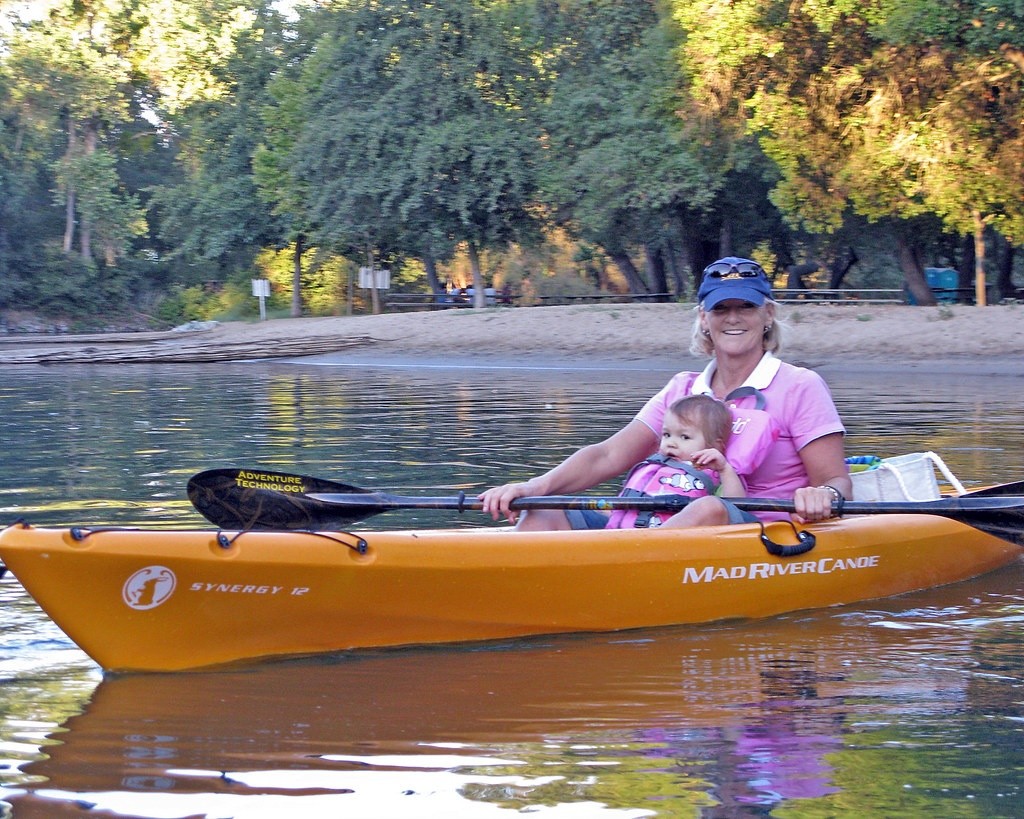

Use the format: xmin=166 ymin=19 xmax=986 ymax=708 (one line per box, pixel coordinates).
xmin=718 ymin=385 xmax=781 ymax=476
xmin=844 ymin=451 xmax=968 ymax=502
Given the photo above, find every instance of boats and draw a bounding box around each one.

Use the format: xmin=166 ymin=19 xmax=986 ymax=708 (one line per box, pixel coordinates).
xmin=1 ymin=476 xmax=1023 ymax=680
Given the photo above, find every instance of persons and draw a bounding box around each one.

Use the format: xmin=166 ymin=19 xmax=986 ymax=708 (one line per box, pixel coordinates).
xmin=476 ymin=256 xmax=853 ymax=532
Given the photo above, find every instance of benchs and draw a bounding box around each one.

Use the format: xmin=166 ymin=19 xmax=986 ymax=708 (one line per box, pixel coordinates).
xmin=383 ymin=294 xmax=522 ymax=307
xmin=770 ymin=289 xmax=904 ymax=303
xmin=931 ymin=287 xmax=977 ymax=301
xmin=534 ymin=293 xmax=672 ymax=305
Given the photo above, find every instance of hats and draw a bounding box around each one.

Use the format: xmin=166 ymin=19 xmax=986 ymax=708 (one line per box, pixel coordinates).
xmin=698 ymin=257 xmax=774 ymax=312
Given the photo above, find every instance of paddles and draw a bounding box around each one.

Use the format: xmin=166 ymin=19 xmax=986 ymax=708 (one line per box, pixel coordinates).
xmin=183 ymin=467 xmax=1024 ymax=550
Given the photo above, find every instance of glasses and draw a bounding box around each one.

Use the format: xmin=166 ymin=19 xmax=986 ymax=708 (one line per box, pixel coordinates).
xmin=704 ymin=261 xmax=765 ymax=277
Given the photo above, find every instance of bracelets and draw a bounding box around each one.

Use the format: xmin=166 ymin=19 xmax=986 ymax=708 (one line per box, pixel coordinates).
xmin=812 ymin=485 xmax=846 ymax=515
